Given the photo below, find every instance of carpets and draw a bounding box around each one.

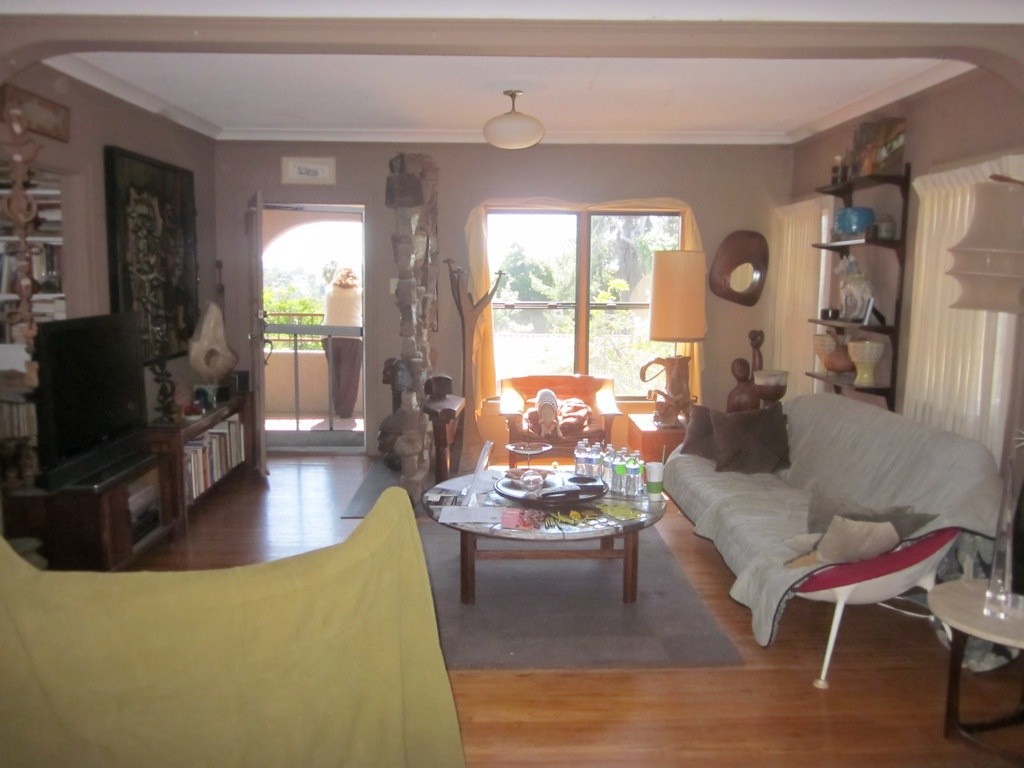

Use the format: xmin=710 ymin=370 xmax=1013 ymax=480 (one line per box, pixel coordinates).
xmin=338 ymin=456 xmax=745 ymax=701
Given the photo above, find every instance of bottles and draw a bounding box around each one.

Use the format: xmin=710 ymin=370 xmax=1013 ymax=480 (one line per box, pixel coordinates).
xmin=621 ymin=447 xmax=627 ymax=455
xmin=575 ymin=441 xmax=585 ymax=456
xmin=581 ymin=438 xmax=592 ymax=454
xmin=575 ymin=444 xmax=587 ymax=475
xmin=603 ymin=449 xmax=616 ymax=491
xmin=589 ymin=447 xmax=601 ymax=478
xmin=626 ymin=453 xmax=639 ymax=496
xmin=831 ymin=154 xmax=844 ymax=183
xmin=605 ymin=443 xmax=614 ymax=455
xmin=634 ymin=450 xmax=640 ymax=463
xmin=612 ymin=451 xmax=627 ymax=493
xmin=592 ymin=442 xmax=604 ymax=462
xmin=638 ymin=460 xmax=645 ymax=491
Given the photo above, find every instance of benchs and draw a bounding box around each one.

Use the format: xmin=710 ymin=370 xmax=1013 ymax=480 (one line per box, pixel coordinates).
xmin=663 ymin=394 xmax=999 ymax=691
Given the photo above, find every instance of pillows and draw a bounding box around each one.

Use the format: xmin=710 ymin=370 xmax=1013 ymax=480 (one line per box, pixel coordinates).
xmin=806 ymin=489 xmax=913 ymax=533
xmin=784 ymin=515 xmax=940 ymax=572
xmin=680 ymin=403 xmax=715 ymax=461
xmin=708 ymin=407 xmax=793 ymax=474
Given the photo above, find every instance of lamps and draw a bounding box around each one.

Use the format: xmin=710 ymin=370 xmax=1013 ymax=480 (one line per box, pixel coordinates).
xmin=639 ymin=249 xmax=710 ymax=426
xmin=482 ymin=88 xmax=547 ymax=151
xmin=942 ymin=172 xmax=1024 ymax=596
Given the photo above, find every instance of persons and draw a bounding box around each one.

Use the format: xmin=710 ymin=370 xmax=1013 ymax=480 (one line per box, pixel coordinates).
xmin=726 ymin=328 xmax=766 ymax=411
xmin=320 ymin=263 xmax=362 ymax=420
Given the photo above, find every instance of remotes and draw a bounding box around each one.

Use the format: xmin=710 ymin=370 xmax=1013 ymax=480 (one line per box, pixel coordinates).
xmin=541 ymin=484 xmax=581 ymax=498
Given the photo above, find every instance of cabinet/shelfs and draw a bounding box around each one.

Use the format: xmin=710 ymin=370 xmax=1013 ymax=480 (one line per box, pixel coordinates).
xmin=0 ymin=388 xmax=264 ymax=575
xmin=803 ymin=158 xmax=911 ymax=414
xmin=0 ymin=178 xmax=67 ymax=454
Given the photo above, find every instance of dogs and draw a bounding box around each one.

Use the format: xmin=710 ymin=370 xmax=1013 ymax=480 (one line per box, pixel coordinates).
xmin=536 ymin=388 xmax=565 ymax=438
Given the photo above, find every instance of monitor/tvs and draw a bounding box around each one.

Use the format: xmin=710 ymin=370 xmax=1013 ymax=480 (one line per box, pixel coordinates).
xmin=33 ymin=308 xmax=156 ymax=491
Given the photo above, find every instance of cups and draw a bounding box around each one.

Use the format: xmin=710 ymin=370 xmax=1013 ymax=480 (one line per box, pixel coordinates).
xmin=877 ymin=222 xmax=896 ymax=239
xmin=645 ymin=462 xmax=664 ymax=501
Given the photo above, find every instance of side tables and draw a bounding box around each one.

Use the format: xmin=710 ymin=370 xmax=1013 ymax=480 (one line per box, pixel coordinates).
xmin=628 ymin=413 xmax=687 ymax=463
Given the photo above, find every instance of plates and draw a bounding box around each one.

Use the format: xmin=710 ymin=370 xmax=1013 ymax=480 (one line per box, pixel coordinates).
xmin=505 ymin=442 xmax=552 ymax=454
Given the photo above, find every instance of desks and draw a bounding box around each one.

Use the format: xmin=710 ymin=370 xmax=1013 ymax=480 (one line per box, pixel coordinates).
xmin=927 ymin=578 xmax=1024 ymax=768
xmin=421 ymin=462 xmax=668 ymax=602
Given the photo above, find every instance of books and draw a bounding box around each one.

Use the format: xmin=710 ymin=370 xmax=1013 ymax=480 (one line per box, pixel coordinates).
xmin=182 ymin=410 xmax=245 ymax=505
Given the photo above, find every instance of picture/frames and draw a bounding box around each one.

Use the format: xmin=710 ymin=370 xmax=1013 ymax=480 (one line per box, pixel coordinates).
xmin=103 ymin=143 xmax=204 ymax=367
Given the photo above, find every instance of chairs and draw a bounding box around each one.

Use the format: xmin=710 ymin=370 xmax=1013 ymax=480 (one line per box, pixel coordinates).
xmin=0 ymin=484 xmax=472 ymax=768
xmin=499 ymin=374 xmax=624 ymax=468
